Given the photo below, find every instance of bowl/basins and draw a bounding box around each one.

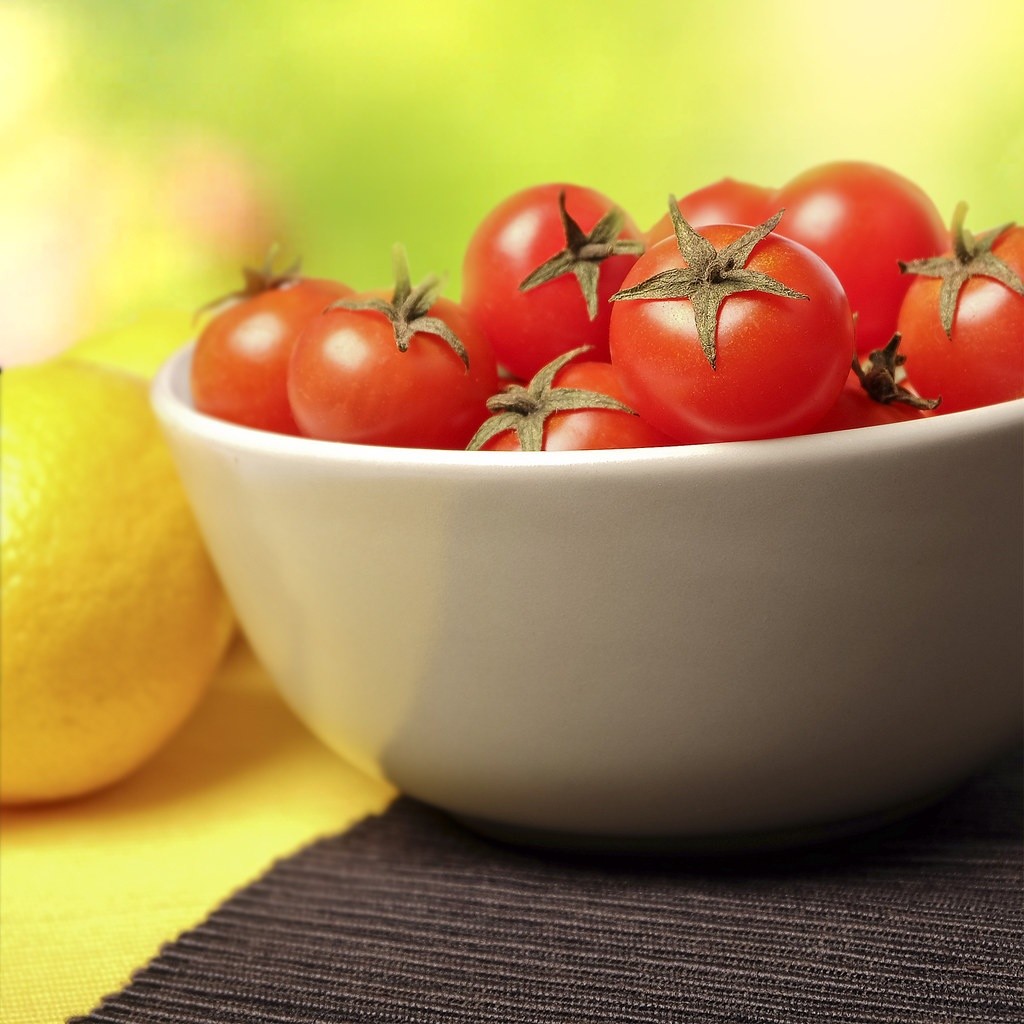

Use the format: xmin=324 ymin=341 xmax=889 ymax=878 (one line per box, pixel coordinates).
xmin=145 ymin=345 xmax=1024 ymax=826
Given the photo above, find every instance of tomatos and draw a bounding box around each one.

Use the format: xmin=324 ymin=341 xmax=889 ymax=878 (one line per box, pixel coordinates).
xmin=181 ymin=152 xmax=1023 ymax=453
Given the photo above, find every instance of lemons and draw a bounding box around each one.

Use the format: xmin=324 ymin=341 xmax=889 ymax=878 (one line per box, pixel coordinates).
xmin=0 ymin=355 xmax=238 ymax=802
